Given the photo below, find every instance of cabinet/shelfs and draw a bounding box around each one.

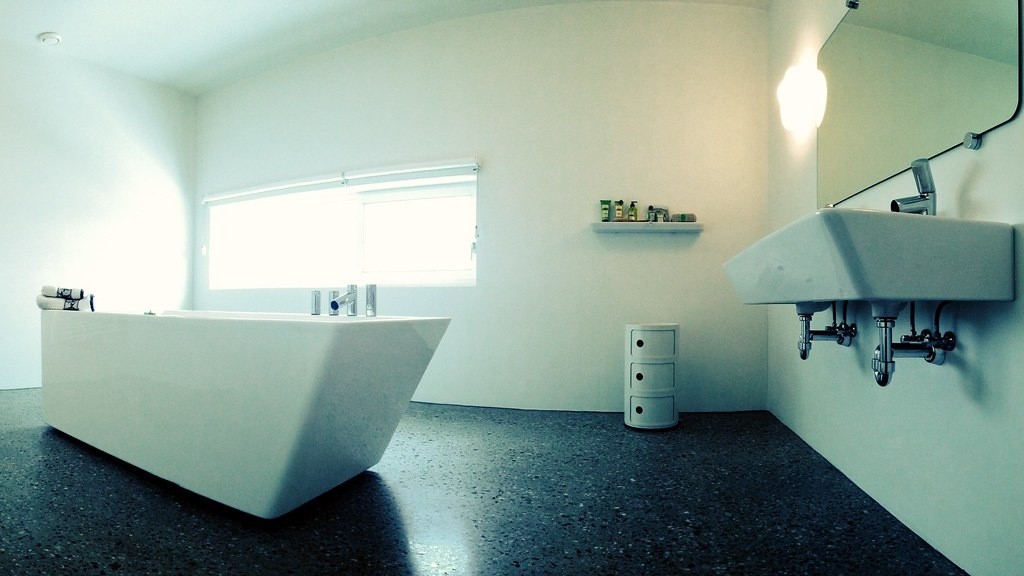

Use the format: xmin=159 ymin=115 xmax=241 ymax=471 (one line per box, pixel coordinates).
xmin=624 ymin=322 xmax=679 ymax=429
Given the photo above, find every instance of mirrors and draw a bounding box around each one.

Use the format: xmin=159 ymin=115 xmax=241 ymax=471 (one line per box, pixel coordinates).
xmin=816 ymin=0 xmax=1020 ymax=209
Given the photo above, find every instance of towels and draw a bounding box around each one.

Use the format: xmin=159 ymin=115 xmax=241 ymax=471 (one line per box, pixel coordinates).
xmin=35 ymin=285 xmax=95 ymax=310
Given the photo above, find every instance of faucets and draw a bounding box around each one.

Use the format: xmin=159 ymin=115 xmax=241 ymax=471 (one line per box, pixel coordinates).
xmin=330 ymin=284 xmax=358 ymax=317
xmin=888 ymin=157 xmax=937 ymax=216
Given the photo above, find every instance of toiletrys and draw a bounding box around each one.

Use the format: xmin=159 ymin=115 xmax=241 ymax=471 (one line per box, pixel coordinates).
xmin=600 ymin=200 xmax=698 ymax=222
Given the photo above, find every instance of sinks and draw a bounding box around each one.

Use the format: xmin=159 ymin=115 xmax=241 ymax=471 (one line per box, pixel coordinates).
xmin=720 ymin=208 xmax=1015 ymax=305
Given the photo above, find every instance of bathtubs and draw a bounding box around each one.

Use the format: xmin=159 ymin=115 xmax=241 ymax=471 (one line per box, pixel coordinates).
xmin=39 ymin=309 xmax=451 ymax=518
